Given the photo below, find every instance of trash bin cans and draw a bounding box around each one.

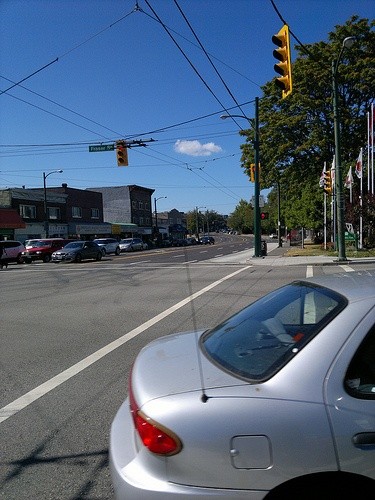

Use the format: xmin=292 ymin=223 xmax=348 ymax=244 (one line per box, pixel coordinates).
xmin=260 ymin=241 xmax=268 ymax=256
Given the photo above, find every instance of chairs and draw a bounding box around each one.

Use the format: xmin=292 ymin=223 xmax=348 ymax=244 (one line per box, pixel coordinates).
xmin=257 ymin=318 xmax=294 ymax=354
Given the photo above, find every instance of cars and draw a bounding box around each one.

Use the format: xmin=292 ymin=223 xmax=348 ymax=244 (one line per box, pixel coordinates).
xmin=24 ymin=239 xmax=38 ymax=247
xmin=92 ymin=239 xmax=121 ymax=255
xmin=52 ymin=241 xmax=104 ymax=263
xmin=163 ymin=237 xmax=197 ymax=248
xmin=117 ymin=238 xmax=143 ymax=252
xmin=110 ymin=269 xmax=375 ymax=500
xmin=200 ymin=237 xmax=215 ymax=245
xmin=270 ymin=234 xmax=278 ymax=239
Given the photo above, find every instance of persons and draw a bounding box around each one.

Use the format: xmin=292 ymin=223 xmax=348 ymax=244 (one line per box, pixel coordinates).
xmin=0 ymin=242 xmax=8 ymax=269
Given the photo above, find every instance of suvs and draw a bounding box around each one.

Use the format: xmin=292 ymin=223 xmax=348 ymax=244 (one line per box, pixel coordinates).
xmin=0 ymin=240 xmax=26 ymax=265
xmin=20 ymin=239 xmax=74 ymax=264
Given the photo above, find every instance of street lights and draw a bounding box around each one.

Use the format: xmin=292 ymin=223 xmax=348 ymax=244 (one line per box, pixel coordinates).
xmin=330 ymin=37 xmax=360 ymax=263
xmin=43 ymin=170 xmax=65 ymax=238
xmin=195 ymin=206 xmax=208 ymax=240
xmin=220 ymin=96 xmax=264 ymax=257
xmin=154 ymin=195 xmax=167 ymax=233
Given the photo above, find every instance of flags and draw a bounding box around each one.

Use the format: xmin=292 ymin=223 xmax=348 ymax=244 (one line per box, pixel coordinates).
xmin=319 ymin=156 xmax=335 ymax=198
xmin=355 ymin=149 xmax=362 ymax=178
xmin=344 ymin=169 xmax=354 ymax=189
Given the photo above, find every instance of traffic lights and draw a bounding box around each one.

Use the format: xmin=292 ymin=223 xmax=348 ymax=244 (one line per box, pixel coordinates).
xmin=117 ymin=140 xmax=128 ymax=166
xmin=272 ymin=25 xmax=295 ymax=100
xmin=323 ymin=171 xmax=332 ymax=192
